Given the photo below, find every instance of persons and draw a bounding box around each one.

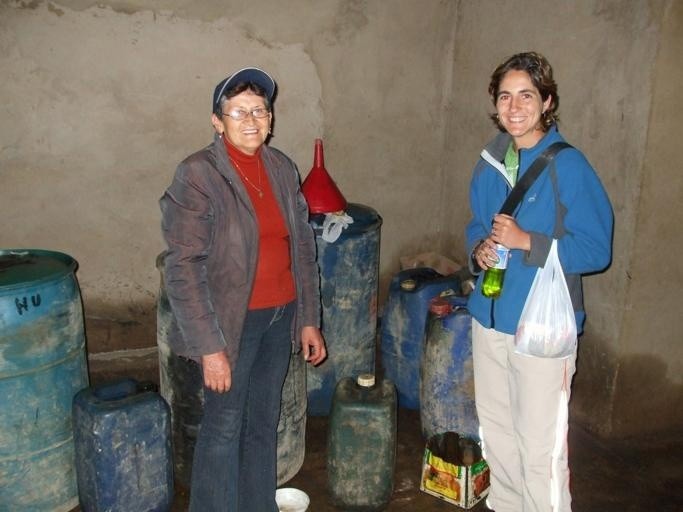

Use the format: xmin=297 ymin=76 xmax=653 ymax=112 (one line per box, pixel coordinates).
xmin=464 ymin=53 xmax=614 ymax=512
xmin=157 ymin=66 xmax=327 ymax=512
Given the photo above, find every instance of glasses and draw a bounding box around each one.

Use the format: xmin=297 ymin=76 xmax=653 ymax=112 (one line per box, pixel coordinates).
xmin=221 ymin=108 xmax=271 ymax=120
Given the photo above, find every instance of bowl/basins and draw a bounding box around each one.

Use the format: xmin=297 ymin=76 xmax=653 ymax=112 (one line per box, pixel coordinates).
xmin=275 ymin=487 xmax=310 ymax=512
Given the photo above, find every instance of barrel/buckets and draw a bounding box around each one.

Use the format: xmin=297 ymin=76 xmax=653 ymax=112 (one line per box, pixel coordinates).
xmin=420 ymin=295 xmax=480 ymax=454
xmin=0 ymin=248 xmax=90 ymax=512
xmin=381 ymin=268 xmax=459 ymax=411
xmin=156 ymin=249 xmax=307 ymax=487
xmin=71 ymin=378 xmax=174 ymax=512
xmin=328 ymin=375 xmax=399 ymax=512
xmin=307 ymin=204 xmax=382 ymax=419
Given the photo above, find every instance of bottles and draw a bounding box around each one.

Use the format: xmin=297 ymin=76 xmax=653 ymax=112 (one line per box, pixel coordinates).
xmin=482 ymin=242 xmax=511 ymax=300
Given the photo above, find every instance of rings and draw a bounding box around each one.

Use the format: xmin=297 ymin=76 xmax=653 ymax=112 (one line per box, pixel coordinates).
xmin=477 ymin=251 xmax=483 ymax=256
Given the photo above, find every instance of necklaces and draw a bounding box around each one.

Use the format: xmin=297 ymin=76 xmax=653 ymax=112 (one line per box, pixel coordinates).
xmin=229 ymin=152 xmax=266 ymax=199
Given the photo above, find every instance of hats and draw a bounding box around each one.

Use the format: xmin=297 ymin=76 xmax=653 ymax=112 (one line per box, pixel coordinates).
xmin=212 ymin=67 xmax=275 ymax=110
